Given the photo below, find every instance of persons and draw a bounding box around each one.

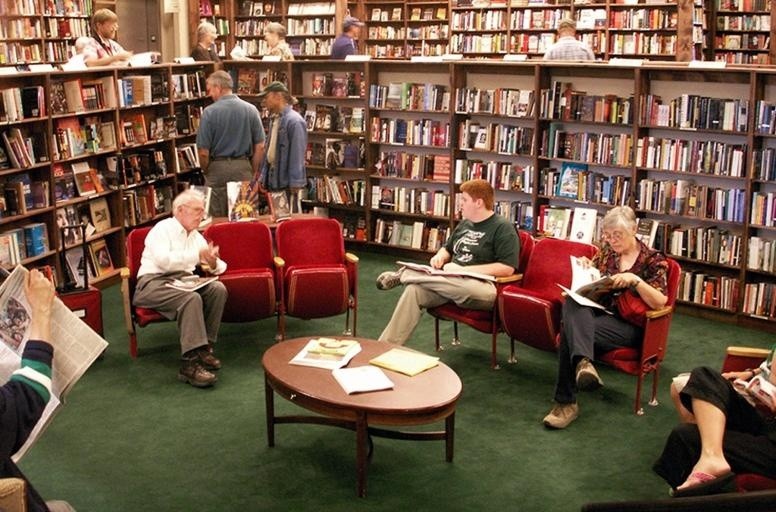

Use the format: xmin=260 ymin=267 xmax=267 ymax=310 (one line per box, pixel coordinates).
xmin=255 ymin=83 xmax=309 ymax=193
xmin=264 ymin=24 xmax=295 ymax=62
xmin=133 ymin=188 xmax=228 ymax=388
xmin=95 ymin=8 xmax=135 ymax=67
xmin=197 ymin=70 xmax=266 ymax=216
xmin=0 ymin=269 xmax=76 ymax=512
xmin=542 ymin=206 xmax=669 ymax=429
xmin=375 ymin=179 xmax=520 ymax=347
xmin=652 ymin=344 xmax=776 ymax=497
xmin=191 ymin=23 xmax=221 ymax=61
xmin=328 ymin=16 xmax=366 ymax=57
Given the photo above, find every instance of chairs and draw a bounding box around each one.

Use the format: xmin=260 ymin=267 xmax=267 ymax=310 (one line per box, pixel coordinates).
xmin=203 ymin=224 xmax=283 ymax=342
xmin=724 ymin=348 xmax=771 ymax=374
xmin=274 ymin=218 xmax=359 ymax=336
xmin=123 ymin=227 xmax=180 ymax=358
xmin=555 ymin=256 xmax=680 ymax=413
xmin=501 ymin=239 xmax=598 ymax=364
xmin=426 ymin=231 xmax=533 ymax=368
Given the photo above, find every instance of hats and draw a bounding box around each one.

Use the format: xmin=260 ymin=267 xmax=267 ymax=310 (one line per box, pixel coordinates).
xmin=255 ymin=81 xmax=288 ymax=98
xmin=558 ymin=18 xmax=577 ymax=28
xmin=343 ymin=17 xmax=365 ymax=28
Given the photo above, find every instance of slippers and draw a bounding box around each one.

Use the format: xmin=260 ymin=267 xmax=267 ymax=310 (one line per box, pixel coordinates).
xmin=672 ymin=472 xmax=735 ymax=496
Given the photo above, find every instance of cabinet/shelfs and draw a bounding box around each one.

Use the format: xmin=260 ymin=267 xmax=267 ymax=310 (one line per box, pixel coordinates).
xmin=454 ymin=62 xmax=537 ymax=232
xmin=218 ymin=61 xmax=367 ymax=246
xmin=537 ymin=62 xmax=634 ymax=256
xmin=114 ymin=64 xmax=214 ymax=226
xmin=368 ymin=61 xmax=454 ymax=260
xmin=636 ymin=63 xmax=775 ymax=327
xmin=0 ymin=0 xmax=96 ymax=67
xmin=0 ymin=62 xmax=123 ymax=295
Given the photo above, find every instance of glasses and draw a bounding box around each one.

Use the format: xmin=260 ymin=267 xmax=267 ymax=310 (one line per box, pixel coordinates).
xmin=182 ymin=205 xmax=204 ymax=213
xmin=600 ymin=232 xmax=623 ymax=241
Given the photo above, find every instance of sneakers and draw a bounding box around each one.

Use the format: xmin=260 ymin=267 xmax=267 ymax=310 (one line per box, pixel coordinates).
xmin=376 ymin=265 xmax=406 ymax=290
xmin=178 ymin=355 xmax=218 ymax=388
xmin=543 ymin=401 xmax=579 ymax=429
xmin=575 ymin=358 xmax=604 ymax=392
xmin=195 ymin=345 xmax=221 ymax=370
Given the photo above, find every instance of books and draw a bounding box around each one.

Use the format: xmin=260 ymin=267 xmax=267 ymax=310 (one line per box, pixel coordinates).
xmin=332 ymin=1 xmax=776 ymax=65
xmin=0 ymin=263 xmax=109 ymax=462
xmin=232 ymin=72 xmax=260 ymax=93
xmin=197 ymin=1 xmax=335 ymax=57
xmin=735 ymin=374 xmax=776 ymax=414
xmin=298 ymin=72 xmax=536 ymax=253
xmin=166 ymin=275 xmax=219 ymax=294
xmin=534 ymin=70 xmax=776 ymax=320
xmin=1 ymin=78 xmax=211 ymax=300
xmin=0 ymin=1 xmax=95 ymax=65
